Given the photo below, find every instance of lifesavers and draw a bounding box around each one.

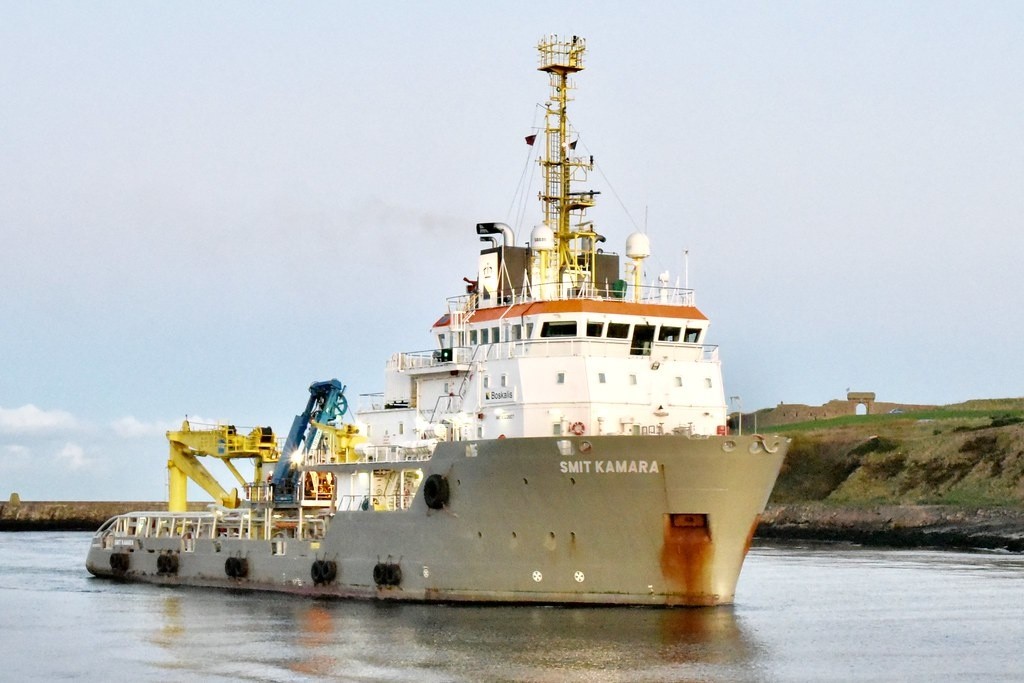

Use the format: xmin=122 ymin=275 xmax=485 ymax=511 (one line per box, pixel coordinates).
xmin=572 ymin=422 xmax=585 ymax=436
xmin=109 ymin=553 xmax=120 ymax=569
xmin=311 ymin=560 xmax=324 ymax=583
xmin=321 ymin=561 xmax=336 ymax=582
xmin=117 ymin=554 xmax=129 ymax=571
xmin=166 ymin=556 xmax=178 ymax=573
xmin=614 ymin=280 xmax=628 ymax=298
xmin=423 ymin=474 xmax=448 ymax=510
xmin=373 ymin=564 xmax=387 ymax=585
xmin=235 ymin=558 xmax=248 ymax=577
xmin=157 ymin=555 xmax=168 ymax=573
xmin=386 ymin=564 xmax=402 ymax=585
xmin=224 ymin=557 xmax=237 ymax=577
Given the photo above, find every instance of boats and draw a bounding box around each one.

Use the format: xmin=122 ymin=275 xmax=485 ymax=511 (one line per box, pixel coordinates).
xmin=85 ymin=29 xmax=793 ymax=608
